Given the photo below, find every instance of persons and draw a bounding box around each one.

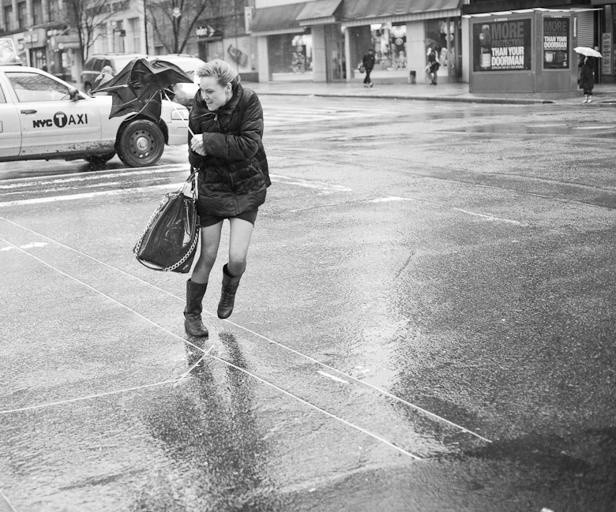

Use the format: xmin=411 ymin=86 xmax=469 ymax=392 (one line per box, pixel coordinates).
xmin=49 ymin=61 xmax=56 ymax=77
xmin=176 ymin=321 xmax=616 ymax=326
xmin=579 ymin=55 xmax=594 ymax=104
xmin=168 ymin=58 xmax=273 ymax=340
xmin=41 ymin=62 xmax=48 ymax=73
xmin=361 ymin=48 xmax=377 ymax=88
xmin=426 ymin=48 xmax=442 ymax=87
xmin=93 ymin=64 xmax=113 ymax=87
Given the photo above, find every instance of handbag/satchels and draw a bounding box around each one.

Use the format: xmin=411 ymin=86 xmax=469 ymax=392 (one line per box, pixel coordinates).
xmin=132 ymin=167 xmax=200 ymax=274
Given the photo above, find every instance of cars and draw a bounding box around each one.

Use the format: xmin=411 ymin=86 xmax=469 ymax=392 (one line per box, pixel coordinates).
xmin=0 ymin=65 xmax=189 ymax=166
xmin=82 ymin=53 xmax=206 ymax=105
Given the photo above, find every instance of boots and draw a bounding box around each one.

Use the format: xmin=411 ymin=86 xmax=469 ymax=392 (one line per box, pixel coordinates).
xmin=183 ymin=280 xmax=209 ymax=338
xmin=216 ymin=264 xmax=242 ymax=319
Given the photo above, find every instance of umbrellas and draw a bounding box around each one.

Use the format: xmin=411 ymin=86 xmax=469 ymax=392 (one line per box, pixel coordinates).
xmin=426 ymin=38 xmax=444 ymax=52
xmin=88 ymin=53 xmax=198 ymax=139
xmin=575 ymin=45 xmax=605 ymax=61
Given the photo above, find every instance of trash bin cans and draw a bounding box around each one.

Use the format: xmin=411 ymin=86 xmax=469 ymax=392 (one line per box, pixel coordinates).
xmin=410 ymin=71 xmax=416 ymax=84
